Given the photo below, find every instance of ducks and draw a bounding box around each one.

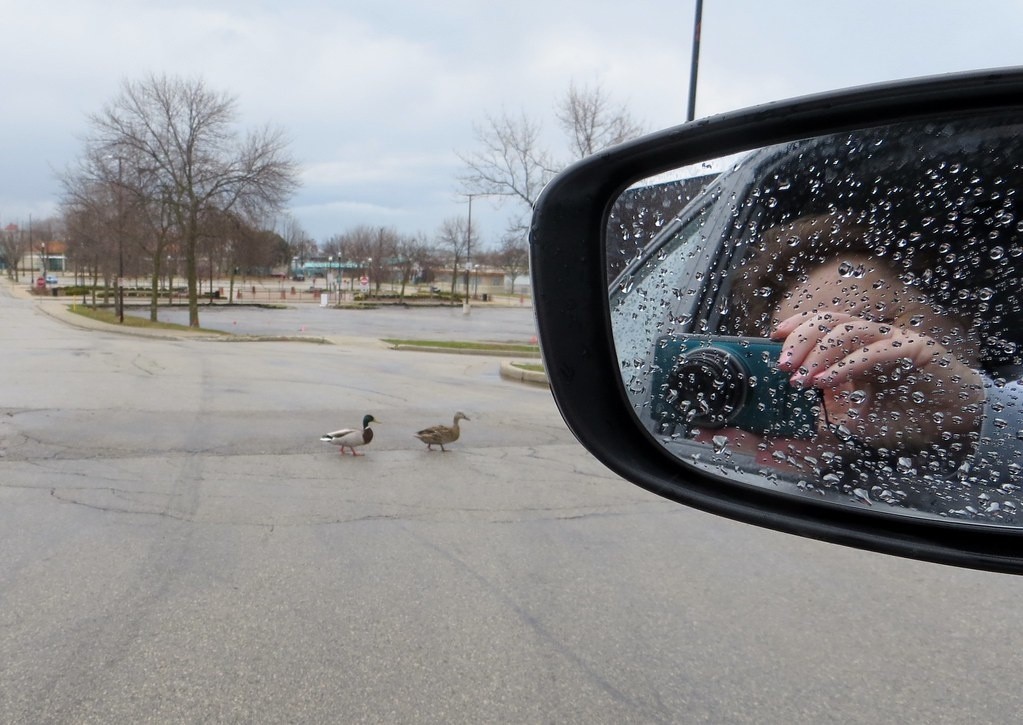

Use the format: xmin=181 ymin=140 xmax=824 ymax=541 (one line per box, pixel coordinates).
xmin=414 ymin=411 xmax=471 ymax=451
xmin=320 ymin=415 xmax=382 ymax=456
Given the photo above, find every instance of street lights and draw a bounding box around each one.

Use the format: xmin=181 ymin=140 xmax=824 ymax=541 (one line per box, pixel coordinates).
xmin=328 ymin=255 xmax=334 ymax=295
xmin=41 ymin=242 xmax=47 ymax=277
xmin=367 ymin=257 xmax=372 ymax=295
xmin=337 ymin=252 xmax=343 ymax=306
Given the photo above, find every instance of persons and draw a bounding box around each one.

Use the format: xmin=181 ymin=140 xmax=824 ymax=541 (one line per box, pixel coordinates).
xmin=696 ymin=211 xmax=1023 ymax=524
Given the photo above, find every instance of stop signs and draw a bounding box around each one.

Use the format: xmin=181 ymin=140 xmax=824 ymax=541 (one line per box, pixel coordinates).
xmin=38 ymin=279 xmax=45 ymax=286
xmin=361 ymin=278 xmax=369 ymax=286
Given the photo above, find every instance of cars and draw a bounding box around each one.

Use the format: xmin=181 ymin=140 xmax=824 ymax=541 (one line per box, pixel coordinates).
xmin=45 ymin=274 xmax=57 ymax=283
xmin=293 ymin=274 xmax=305 ymax=281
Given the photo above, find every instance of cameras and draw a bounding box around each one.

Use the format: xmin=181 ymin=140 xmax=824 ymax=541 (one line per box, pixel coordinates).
xmin=648 ymin=332 xmax=822 ymax=441
